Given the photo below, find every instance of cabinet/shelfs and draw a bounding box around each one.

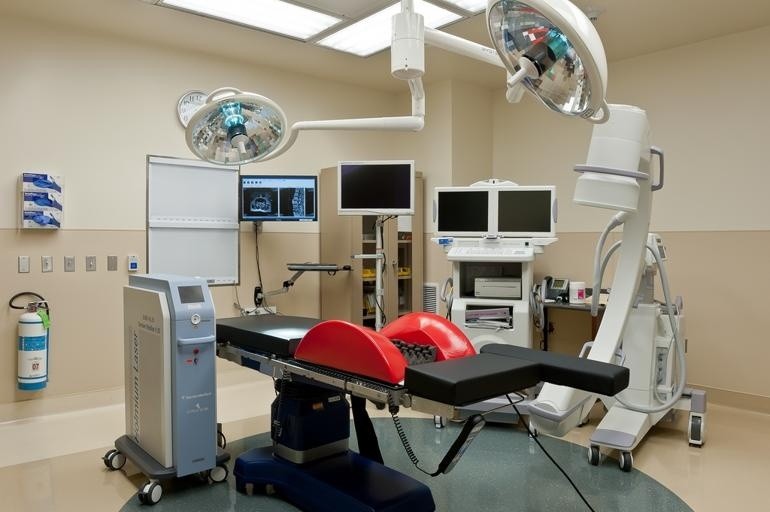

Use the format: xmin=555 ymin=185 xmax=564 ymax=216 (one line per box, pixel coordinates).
xmin=318 ymin=166 xmax=425 ymax=330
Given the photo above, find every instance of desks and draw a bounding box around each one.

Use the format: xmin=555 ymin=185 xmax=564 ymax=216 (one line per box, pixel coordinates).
xmin=537 ymin=301 xmax=605 ymax=351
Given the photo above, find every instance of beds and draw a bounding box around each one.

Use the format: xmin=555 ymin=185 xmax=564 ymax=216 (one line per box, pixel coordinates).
xmin=214 ymin=311 xmax=630 ymax=512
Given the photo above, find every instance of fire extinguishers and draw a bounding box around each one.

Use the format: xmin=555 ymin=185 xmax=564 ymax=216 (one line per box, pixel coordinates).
xmin=10 ymin=291 xmax=51 ymax=391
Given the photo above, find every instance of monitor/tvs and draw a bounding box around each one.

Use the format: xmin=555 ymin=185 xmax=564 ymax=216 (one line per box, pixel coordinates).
xmin=433 ymin=187 xmax=495 ymax=238
xmin=338 ymin=160 xmax=414 ymax=215
xmin=496 ymin=186 xmax=557 ymax=238
xmin=239 ymin=175 xmax=319 ymax=221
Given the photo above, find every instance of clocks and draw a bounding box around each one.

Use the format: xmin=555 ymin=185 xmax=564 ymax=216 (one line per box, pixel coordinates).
xmin=177 ymin=90 xmax=208 ymax=128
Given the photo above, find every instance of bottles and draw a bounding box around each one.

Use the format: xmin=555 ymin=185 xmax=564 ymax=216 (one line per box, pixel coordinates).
xmin=569 ymin=281 xmax=586 ymax=304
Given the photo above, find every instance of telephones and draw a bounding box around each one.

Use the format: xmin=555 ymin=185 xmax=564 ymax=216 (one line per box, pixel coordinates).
xmin=541 ymin=276 xmax=569 ymax=299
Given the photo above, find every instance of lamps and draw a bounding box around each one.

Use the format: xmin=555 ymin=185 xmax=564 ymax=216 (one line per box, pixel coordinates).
xmin=186 ymin=0 xmax=612 ymax=167
